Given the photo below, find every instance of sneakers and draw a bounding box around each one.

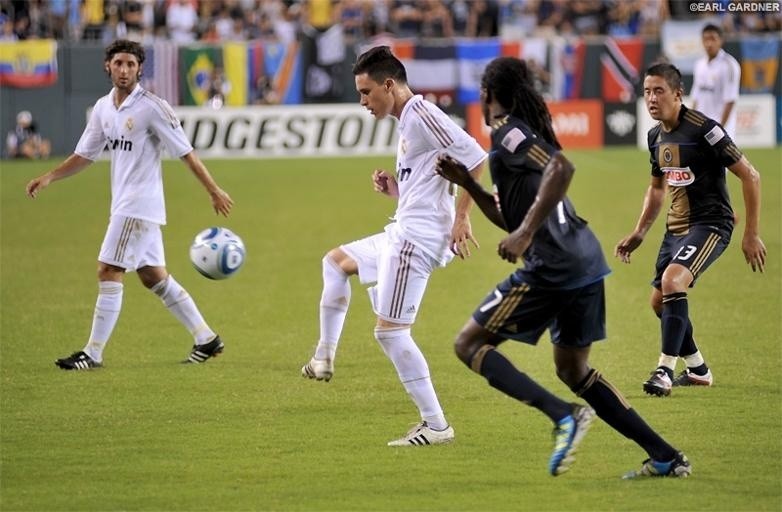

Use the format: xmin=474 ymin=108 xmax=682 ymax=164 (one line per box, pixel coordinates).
xmin=643 ymin=369 xmax=674 ymax=398
xmin=181 ymin=335 xmax=226 ymax=365
xmin=548 ymin=403 xmax=596 ymax=476
xmin=672 ymin=368 xmax=713 ymax=387
xmin=53 ymin=350 xmax=104 ymax=370
xmin=387 ymin=420 xmax=455 ymax=448
xmin=301 ymin=355 xmax=335 ymax=382
xmin=621 ymin=450 xmax=692 ymax=481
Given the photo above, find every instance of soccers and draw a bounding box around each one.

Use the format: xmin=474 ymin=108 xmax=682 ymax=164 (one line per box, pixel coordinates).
xmin=191 ymin=227 xmax=245 ymax=278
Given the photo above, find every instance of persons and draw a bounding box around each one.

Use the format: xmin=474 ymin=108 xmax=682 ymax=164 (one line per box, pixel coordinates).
xmin=435 ymin=56 xmax=691 ymax=481
xmin=250 ymin=76 xmax=282 ymax=104
xmin=7 ymin=109 xmax=55 ymax=159
xmin=689 ymin=23 xmax=741 ymax=174
xmin=299 ymin=45 xmax=490 ymax=446
xmin=526 ymin=55 xmax=558 ymax=103
xmin=24 ymin=38 xmax=236 ymax=372
xmin=201 ymin=63 xmax=231 ymax=105
xmin=302 ymin=60 xmax=340 ymax=103
xmin=614 ymin=62 xmax=769 ymax=394
